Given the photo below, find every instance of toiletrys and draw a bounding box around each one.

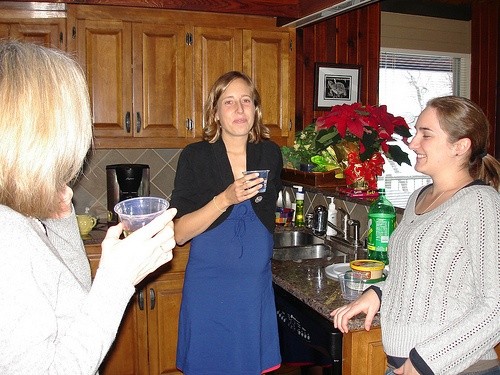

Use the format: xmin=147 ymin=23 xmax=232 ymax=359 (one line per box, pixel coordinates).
xmin=325 ymin=197 xmax=337 ymax=236
xmin=292 ymin=185 xmax=305 ymax=228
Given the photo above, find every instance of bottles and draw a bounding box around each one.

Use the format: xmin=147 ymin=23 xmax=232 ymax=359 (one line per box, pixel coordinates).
xmin=313 ymin=205 xmax=327 ymax=236
xmin=366 ymin=188 xmax=397 ymax=265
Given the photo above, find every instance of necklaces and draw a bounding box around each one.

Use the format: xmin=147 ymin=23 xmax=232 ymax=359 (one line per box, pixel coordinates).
xmin=411 ymin=191 xmax=443 ymax=224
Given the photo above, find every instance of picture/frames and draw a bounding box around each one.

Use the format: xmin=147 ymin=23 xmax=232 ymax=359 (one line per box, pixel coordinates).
xmin=313 ymin=62 xmax=362 ymax=110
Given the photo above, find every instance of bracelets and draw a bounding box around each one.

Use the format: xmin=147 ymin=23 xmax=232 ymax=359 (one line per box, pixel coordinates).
xmin=213 ymin=196 xmax=227 ymax=212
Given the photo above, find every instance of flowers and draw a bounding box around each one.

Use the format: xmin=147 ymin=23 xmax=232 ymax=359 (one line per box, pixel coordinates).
xmin=281 ymin=100 xmax=413 ymax=190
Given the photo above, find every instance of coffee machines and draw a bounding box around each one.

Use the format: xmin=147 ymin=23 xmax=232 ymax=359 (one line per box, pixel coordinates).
xmin=105 ymin=164 xmax=151 ymax=239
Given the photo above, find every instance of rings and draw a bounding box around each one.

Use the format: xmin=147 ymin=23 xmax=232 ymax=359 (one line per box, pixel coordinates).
xmin=160 ymin=245 xmax=167 ymax=253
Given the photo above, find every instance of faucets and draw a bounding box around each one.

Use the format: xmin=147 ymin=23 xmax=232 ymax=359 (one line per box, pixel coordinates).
xmin=309 ymin=207 xmax=351 ymax=240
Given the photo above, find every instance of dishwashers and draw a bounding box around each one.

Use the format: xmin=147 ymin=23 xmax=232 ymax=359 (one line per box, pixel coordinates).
xmin=272 ymin=282 xmax=342 ymax=374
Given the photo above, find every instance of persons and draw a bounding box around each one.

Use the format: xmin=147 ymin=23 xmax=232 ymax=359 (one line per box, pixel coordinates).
xmin=170 ymin=72 xmax=283 ymax=375
xmin=0 ymin=42 xmax=178 ymax=375
xmin=331 ymin=96 xmax=500 ymax=375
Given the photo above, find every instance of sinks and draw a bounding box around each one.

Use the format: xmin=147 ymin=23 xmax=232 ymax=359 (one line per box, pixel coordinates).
xmin=272 ymin=244 xmax=338 ymax=262
xmin=273 ymin=231 xmax=324 ymax=248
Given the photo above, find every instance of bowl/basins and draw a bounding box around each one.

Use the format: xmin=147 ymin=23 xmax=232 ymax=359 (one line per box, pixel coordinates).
xmin=275 ymin=208 xmax=294 ymax=225
xmin=350 ymin=259 xmax=385 ymax=282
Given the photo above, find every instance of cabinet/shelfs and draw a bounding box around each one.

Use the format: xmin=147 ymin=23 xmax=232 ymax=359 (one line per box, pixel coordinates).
xmin=66 ymin=0 xmax=192 ymax=150
xmin=83 ymin=242 xmax=191 ymax=375
xmin=0 ymin=0 xmax=66 ymax=56
xmin=193 ymin=0 xmax=294 ymax=141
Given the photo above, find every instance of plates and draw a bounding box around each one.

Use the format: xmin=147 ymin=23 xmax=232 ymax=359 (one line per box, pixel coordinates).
xmin=324 ymin=262 xmax=350 ymax=279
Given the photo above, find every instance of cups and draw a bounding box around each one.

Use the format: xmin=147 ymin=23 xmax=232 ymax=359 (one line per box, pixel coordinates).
xmin=113 ymin=196 xmax=170 ymax=236
xmin=338 ymin=272 xmax=366 ymax=301
xmin=242 ymin=169 xmax=271 ymax=193
xmin=75 ymin=214 xmax=97 ymax=236
